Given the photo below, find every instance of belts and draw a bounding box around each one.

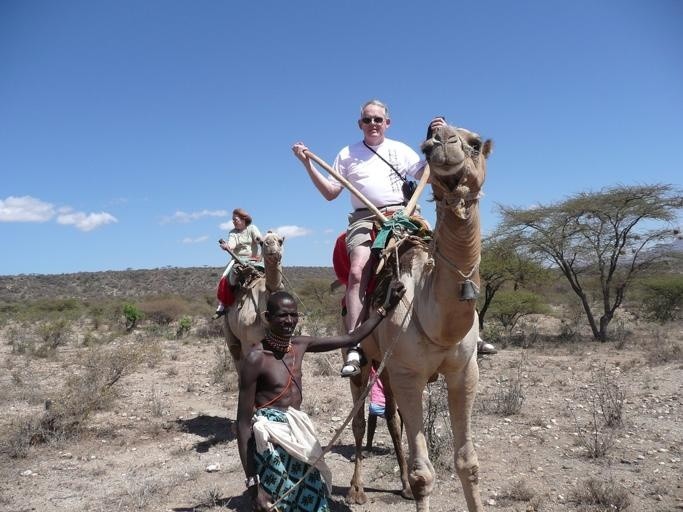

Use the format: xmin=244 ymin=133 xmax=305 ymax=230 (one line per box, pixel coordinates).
xmin=355 ymin=202 xmax=404 ymax=211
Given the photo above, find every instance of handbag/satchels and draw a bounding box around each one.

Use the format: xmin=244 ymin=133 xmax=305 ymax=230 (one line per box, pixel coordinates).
xmin=402 ymin=179 xmax=416 ymax=200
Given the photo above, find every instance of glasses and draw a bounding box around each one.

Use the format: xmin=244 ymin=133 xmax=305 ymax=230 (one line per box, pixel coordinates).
xmin=361 ymin=117 xmax=384 ymax=124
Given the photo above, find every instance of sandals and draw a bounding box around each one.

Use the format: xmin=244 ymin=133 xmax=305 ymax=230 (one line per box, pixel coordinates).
xmin=476 ymin=338 xmax=498 ymax=355
xmin=339 ymin=346 xmax=368 ymax=377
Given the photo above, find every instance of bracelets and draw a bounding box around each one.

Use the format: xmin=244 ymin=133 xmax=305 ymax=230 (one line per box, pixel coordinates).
xmin=244 ymin=473 xmax=261 ymax=489
xmin=376 ymin=306 xmax=387 ymax=318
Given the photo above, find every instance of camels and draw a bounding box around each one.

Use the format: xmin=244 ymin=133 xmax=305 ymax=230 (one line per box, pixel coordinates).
xmin=221 ymin=228 xmax=287 ymax=376
xmin=346 ymin=117 xmax=496 ymax=512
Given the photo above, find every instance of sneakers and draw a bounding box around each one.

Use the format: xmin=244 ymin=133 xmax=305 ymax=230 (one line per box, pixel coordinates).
xmin=210 ymin=309 xmax=226 ymax=320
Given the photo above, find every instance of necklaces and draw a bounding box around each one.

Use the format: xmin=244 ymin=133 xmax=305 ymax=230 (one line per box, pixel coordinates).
xmin=264 ymin=329 xmax=292 ymax=354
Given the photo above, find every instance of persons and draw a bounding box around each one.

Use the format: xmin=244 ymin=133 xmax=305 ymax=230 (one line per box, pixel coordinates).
xmin=210 ymin=207 xmax=261 ymax=320
xmin=290 ymin=97 xmax=498 ymax=378
xmin=233 ymin=280 xmax=407 ymax=512
